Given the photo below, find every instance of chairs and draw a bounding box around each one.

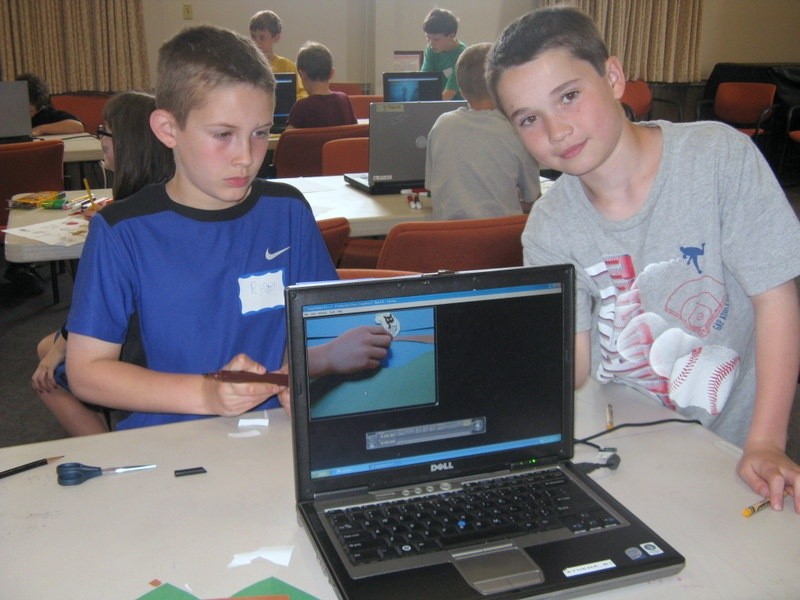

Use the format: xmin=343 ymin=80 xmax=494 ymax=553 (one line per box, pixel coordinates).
xmin=0 ymin=80 xmax=800 ymax=280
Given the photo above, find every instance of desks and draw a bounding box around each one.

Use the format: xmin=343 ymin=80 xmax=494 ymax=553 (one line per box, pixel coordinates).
xmin=0 ymin=372 xmax=800 ymax=600
xmin=34 ymin=133 xmax=104 ymax=190
xmin=4 ymin=171 xmax=559 ymax=261
xmin=268 ymin=119 xmax=369 ymax=177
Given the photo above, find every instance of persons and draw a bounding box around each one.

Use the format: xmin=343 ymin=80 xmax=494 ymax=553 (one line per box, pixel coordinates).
xmin=14 ymin=71 xmax=85 ymax=137
xmin=485 ymin=6 xmax=800 ymax=516
xmin=33 ymin=92 xmax=175 ymax=438
xmin=248 ymin=11 xmax=309 ymax=102
xmin=425 ymin=43 xmax=542 ymax=222
xmin=415 ymin=8 xmax=474 ymax=103
xmin=284 ymin=43 xmax=356 ymax=127
xmin=307 ymin=324 xmax=394 ymax=377
xmin=65 ymin=26 xmax=342 ymax=431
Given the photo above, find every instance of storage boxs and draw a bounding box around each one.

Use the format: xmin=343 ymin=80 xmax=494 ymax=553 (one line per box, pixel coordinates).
xmin=7 ymin=192 xmax=66 ymax=210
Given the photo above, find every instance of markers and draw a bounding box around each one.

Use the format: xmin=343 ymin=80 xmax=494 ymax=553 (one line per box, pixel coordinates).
xmin=400 ymin=188 xmax=426 ymax=193
xmin=414 ymin=197 xmax=422 ymax=209
xmin=407 ymin=194 xmax=415 ymax=208
xmin=418 ymin=191 xmax=431 ymax=197
xmin=61 ymin=194 xmax=107 ymax=209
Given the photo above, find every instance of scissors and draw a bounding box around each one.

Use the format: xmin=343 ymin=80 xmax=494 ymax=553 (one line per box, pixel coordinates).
xmin=56 ymin=461 xmax=156 ymax=485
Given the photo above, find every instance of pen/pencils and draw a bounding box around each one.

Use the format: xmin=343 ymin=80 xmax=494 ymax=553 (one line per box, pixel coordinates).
xmin=84 ymin=179 xmax=95 ymax=209
xmin=203 ymin=371 xmax=289 ymax=387
xmin=0 ymin=455 xmax=63 ymax=478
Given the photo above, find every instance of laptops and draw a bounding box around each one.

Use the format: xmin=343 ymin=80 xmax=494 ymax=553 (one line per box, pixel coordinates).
xmin=269 ymin=72 xmax=297 ymax=134
xmin=382 ymin=73 xmax=442 ymax=103
xmin=0 ymin=81 xmax=33 ymax=144
xmin=282 ymin=263 xmax=685 ymax=599
xmin=343 ymin=101 xmax=471 ymax=194
xmin=394 ymin=51 xmax=424 ymax=72
